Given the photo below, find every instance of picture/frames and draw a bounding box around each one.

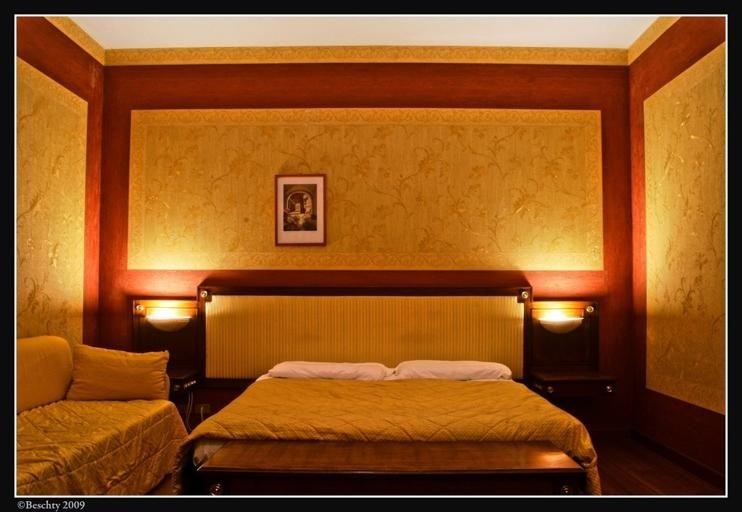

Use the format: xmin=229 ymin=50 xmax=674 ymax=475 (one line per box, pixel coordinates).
xmin=274 ymin=174 xmax=326 ymax=247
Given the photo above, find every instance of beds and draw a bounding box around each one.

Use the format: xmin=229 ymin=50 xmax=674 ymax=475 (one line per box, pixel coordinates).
xmin=187 ymin=372 xmax=589 ymax=494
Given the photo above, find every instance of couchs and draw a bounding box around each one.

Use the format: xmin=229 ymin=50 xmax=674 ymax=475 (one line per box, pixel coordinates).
xmin=17 ymin=337 xmax=188 ymax=494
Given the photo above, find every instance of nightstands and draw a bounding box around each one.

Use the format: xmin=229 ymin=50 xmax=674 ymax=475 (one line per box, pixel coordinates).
xmin=532 ymin=369 xmax=620 ymax=437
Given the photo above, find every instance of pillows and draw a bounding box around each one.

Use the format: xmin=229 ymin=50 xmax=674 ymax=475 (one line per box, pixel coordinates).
xmin=269 ymin=360 xmax=513 ymax=380
xmin=64 ymin=342 xmax=170 ymax=400
